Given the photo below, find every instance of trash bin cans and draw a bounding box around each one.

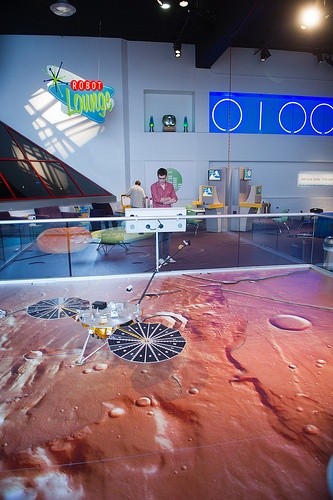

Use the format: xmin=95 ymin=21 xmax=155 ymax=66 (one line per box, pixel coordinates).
xmin=323 ymin=236 xmax=333 ymax=271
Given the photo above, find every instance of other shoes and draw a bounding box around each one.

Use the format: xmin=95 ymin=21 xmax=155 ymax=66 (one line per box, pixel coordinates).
xmin=165 ymin=256 xmax=176 ymax=263
xmin=159 ymin=259 xmax=169 ymax=266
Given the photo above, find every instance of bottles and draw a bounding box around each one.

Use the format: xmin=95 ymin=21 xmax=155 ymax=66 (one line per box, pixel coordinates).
xmin=149 ymin=198 xmax=153 ymax=208
xmin=183 ymin=116 xmax=188 ymax=133
xmin=149 ymin=116 xmax=154 ymax=132
xmin=144 ymin=197 xmax=149 ymax=208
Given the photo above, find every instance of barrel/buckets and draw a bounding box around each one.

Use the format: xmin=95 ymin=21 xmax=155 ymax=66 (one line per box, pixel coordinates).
xmin=323 ymin=236 xmax=333 ymax=272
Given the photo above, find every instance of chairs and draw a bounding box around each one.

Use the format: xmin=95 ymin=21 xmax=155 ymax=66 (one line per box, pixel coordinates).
xmin=270 ymin=208 xmax=291 ymax=236
xmin=186 ymin=209 xmax=204 ymax=235
xmin=296 ymin=210 xmax=305 ymax=232
xmin=295 ymin=211 xmax=333 ymax=263
xmin=27 ymin=215 xmax=43 ymax=242
xmin=304 ymin=207 xmax=324 ymax=228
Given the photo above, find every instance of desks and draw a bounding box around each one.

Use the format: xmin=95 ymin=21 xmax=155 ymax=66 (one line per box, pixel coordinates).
xmin=193 ymin=210 xmax=205 ymax=215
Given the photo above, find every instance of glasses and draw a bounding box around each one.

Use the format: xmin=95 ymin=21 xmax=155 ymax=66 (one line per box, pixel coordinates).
xmin=158 ymin=175 xmax=166 ymax=180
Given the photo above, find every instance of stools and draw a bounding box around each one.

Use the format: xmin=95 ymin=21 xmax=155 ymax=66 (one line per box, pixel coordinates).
xmin=89 ymin=226 xmax=156 ymax=256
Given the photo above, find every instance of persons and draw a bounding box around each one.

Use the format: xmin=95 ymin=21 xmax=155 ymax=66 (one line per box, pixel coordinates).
xmin=150 ymin=168 xmax=178 ymax=265
xmin=126 ymin=181 xmax=146 ymax=208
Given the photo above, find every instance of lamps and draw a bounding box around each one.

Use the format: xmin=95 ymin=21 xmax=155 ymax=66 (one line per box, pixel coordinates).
xmin=48 ymin=0 xmax=77 ymax=18
xmin=173 ymin=39 xmax=181 ymax=53
xmin=175 ymin=53 xmax=181 ymax=58
xmin=317 ymin=53 xmax=324 ymax=64
xmin=259 ymin=50 xmax=266 ymax=62
xmin=324 ymin=53 xmax=333 ymax=67
xmin=263 ymin=47 xmax=272 ymax=59
xmin=159 ymin=0 xmax=173 ymax=10
